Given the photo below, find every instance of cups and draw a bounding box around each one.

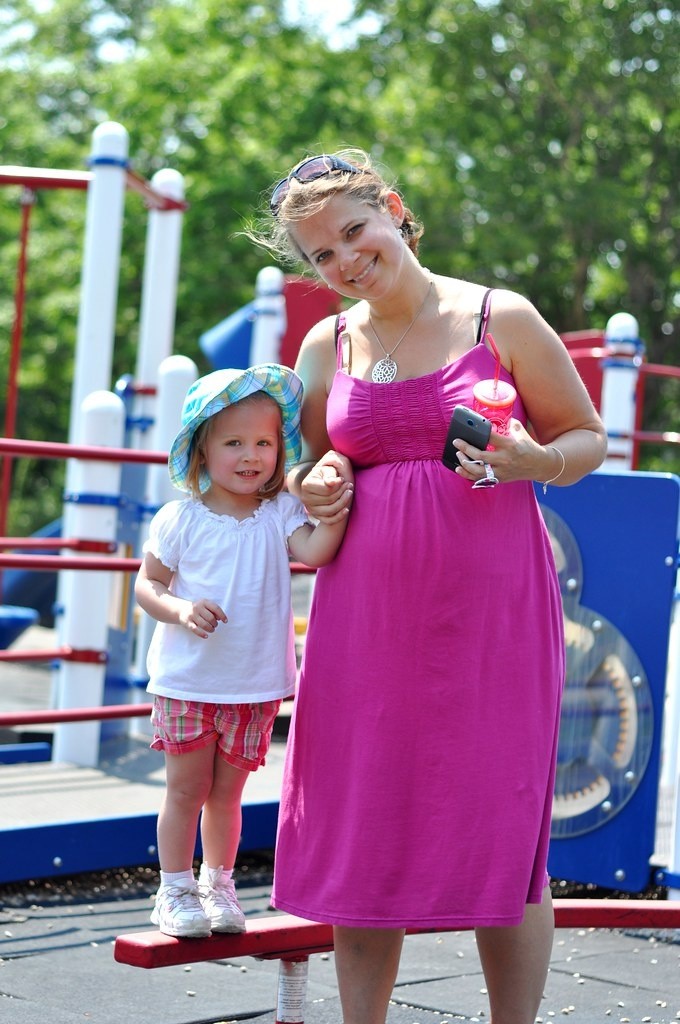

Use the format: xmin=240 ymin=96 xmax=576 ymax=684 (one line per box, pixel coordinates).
xmin=474 ymin=379 xmax=518 ymax=467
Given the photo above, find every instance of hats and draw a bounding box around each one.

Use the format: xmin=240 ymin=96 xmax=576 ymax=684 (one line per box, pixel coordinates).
xmin=168 ymin=363 xmax=304 ymax=495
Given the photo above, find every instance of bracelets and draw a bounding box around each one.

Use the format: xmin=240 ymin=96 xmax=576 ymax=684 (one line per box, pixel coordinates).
xmin=536 ymin=445 xmax=565 ymax=494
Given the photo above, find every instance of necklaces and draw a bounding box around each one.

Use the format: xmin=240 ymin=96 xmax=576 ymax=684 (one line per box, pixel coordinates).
xmin=369 ymin=282 xmax=432 ymax=384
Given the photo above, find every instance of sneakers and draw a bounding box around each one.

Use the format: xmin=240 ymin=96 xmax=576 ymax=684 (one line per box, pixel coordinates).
xmin=199 ymin=861 xmax=246 ymax=934
xmin=150 ymin=878 xmax=212 ymax=938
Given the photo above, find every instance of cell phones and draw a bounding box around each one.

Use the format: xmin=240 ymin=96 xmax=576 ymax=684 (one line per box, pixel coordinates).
xmin=443 ymin=405 xmax=492 ymax=473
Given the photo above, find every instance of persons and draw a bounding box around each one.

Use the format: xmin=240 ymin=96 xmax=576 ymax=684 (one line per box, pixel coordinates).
xmin=134 ymin=363 xmax=353 ymax=938
xmin=266 ymin=145 xmax=611 ymax=1024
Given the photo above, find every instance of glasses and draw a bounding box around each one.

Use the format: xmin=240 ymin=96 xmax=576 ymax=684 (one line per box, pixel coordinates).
xmin=270 ymin=156 xmax=366 ymax=216
